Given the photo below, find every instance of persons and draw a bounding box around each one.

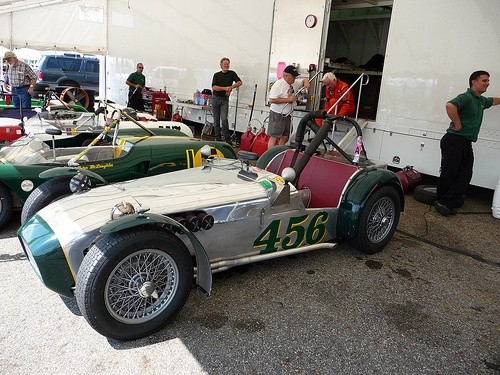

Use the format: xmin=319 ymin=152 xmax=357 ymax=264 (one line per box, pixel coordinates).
xmin=212 ymin=58 xmax=243 ymax=144
xmin=268 ymin=64 xmax=311 ymax=150
xmin=126 ymin=63 xmax=145 ymax=111
xmin=3 ymin=51 xmax=37 ymax=108
xmin=314 ymin=72 xmax=355 ymax=129
xmin=434 ymin=70 xmax=499 ymax=217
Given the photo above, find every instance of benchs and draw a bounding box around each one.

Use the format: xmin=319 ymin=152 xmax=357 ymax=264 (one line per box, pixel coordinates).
xmin=277 ymin=150 xmax=357 ymax=208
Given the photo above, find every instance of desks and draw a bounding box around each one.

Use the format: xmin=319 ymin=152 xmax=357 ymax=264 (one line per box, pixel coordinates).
xmin=166 ymin=101 xmax=214 ymax=139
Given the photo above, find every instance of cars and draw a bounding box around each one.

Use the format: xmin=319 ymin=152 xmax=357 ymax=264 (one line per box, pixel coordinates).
xmin=18 ymin=110 xmax=404 ymax=342
xmin=0 ymin=97 xmax=237 ymax=230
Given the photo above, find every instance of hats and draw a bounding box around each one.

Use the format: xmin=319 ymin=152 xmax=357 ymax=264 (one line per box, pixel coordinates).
xmin=283 ymin=65 xmax=300 ymax=77
xmin=2 ymin=51 xmax=16 ymax=59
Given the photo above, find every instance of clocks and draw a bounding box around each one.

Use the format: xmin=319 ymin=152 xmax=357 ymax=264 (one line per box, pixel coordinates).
xmin=305 ymin=14 xmax=317 ymax=28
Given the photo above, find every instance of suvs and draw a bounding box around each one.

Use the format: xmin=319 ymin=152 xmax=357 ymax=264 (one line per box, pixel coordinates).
xmin=33 ymin=52 xmax=99 ymax=99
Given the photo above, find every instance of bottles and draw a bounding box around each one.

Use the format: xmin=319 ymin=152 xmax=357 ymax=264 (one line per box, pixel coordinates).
xmin=252 ymin=127 xmax=270 ymax=156
xmin=240 ymin=127 xmax=255 ymax=152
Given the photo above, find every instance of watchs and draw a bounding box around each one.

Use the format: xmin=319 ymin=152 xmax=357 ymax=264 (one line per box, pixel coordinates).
xmin=30 ymin=84 xmax=34 ymax=88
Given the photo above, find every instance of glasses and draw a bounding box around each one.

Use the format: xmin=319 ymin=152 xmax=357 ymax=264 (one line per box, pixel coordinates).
xmin=138 ymin=67 xmax=143 ymax=69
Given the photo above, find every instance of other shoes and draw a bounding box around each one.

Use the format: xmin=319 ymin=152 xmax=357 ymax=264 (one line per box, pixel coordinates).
xmin=435 ymin=204 xmax=457 ymax=217
